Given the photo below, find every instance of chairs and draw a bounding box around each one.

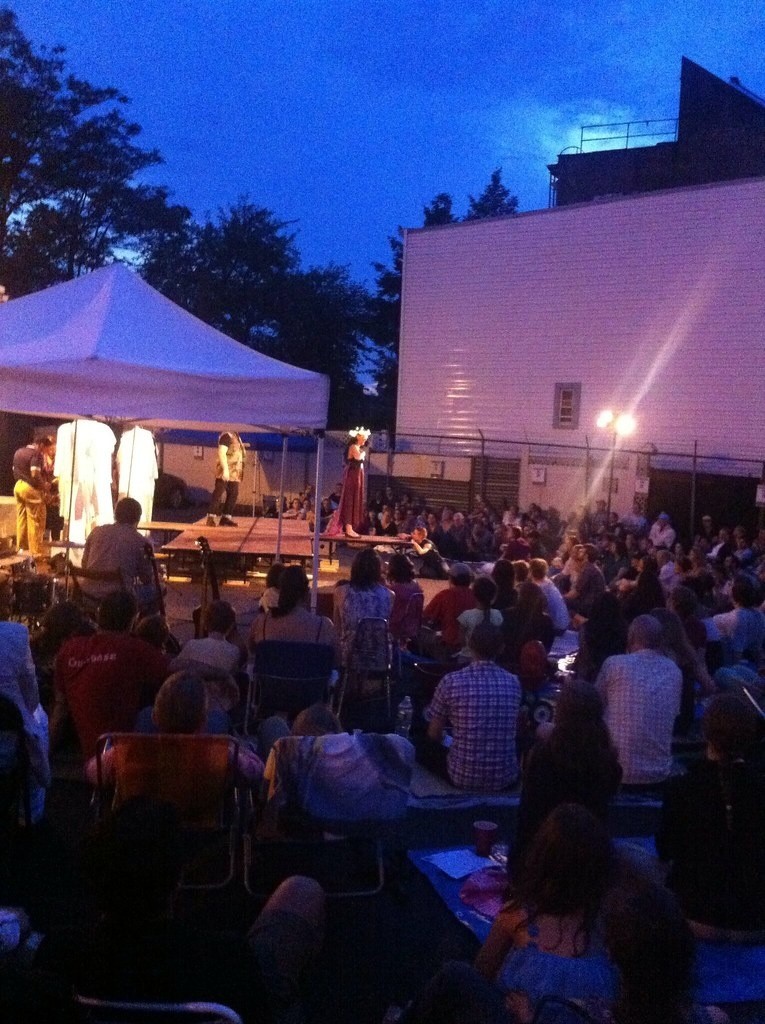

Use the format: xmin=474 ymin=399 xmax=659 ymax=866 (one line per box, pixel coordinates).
xmin=0 ymin=592 xmax=765 ymax=1024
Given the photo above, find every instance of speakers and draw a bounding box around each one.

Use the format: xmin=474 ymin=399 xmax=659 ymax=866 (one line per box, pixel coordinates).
xmin=263 ymin=496 xmax=287 ymax=518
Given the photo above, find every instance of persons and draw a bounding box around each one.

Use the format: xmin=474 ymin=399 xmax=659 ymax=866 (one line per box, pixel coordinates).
xmin=39 ymin=434 xmax=65 ymax=541
xmin=658 ymin=692 xmax=765 ymax=946
xmin=515 ymin=680 xmax=621 ymax=846
xmin=0 ymin=483 xmax=764 ymax=1024
xmin=207 ymin=429 xmax=246 ymax=528
xmin=248 ymin=565 xmax=343 ymax=725
xmin=336 ymin=428 xmax=371 ymax=540
xmin=12 ymin=434 xmax=51 ymax=564
xmin=411 ymin=624 xmax=523 ymax=791
xmin=81 ymin=498 xmax=167 ymax=619
xmin=594 ymin=614 xmax=681 ymax=792
xmin=473 ymin=806 xmax=639 ymax=1023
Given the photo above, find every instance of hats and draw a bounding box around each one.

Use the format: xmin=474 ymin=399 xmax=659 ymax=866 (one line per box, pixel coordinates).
xmin=656 ymin=513 xmax=670 ymax=522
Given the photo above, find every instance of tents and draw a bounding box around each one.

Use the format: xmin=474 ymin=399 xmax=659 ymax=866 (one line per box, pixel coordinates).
xmin=0 ymin=262 xmax=330 ymax=616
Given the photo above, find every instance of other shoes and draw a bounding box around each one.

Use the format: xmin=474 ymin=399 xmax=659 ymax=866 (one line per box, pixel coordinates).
xmin=206 ymin=516 xmax=239 ymax=528
